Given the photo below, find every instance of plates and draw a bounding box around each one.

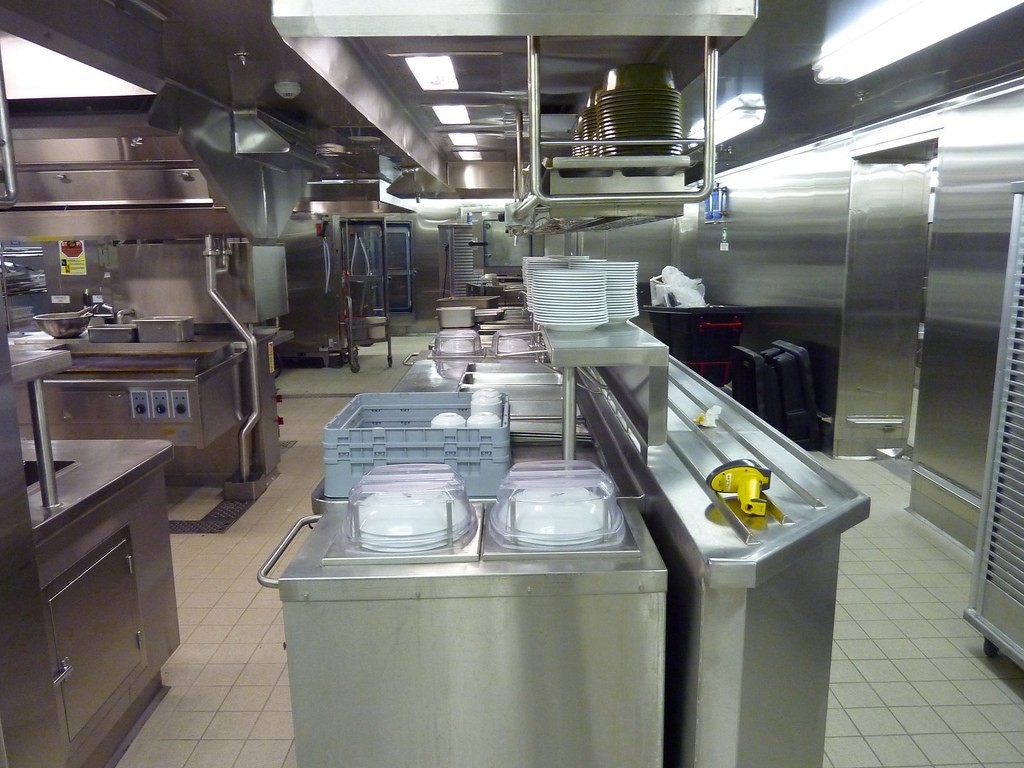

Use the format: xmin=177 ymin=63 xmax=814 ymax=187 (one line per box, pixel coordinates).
xmin=521 ymin=254 xmax=640 ymax=332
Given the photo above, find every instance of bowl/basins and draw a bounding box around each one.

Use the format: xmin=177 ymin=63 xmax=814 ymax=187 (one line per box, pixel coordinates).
xmin=86 ymin=324 xmax=138 ymax=343
xmin=498 ymin=340 xmax=530 ymax=352
xmin=565 ymin=65 xmax=684 ymax=156
xmin=499 ymin=488 xmax=614 ymax=548
xmin=436 ymin=305 xmax=476 ymax=328
xmin=32 ymin=313 xmax=93 ymax=338
xmin=435 ymin=296 xmax=500 ymax=310
xmin=431 ymin=386 xmax=503 ymax=429
xmin=441 ymin=339 xmax=473 ymax=353
xmin=132 ymin=316 xmax=194 ymax=342
xmin=358 ymin=491 xmax=470 ymax=554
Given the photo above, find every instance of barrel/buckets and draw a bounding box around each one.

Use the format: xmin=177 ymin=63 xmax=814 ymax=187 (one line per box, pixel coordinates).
xmin=366 ymin=316 xmax=386 ymax=338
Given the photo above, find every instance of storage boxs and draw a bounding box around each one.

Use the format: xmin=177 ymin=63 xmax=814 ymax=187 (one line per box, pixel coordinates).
xmin=345 ymin=316 xmax=386 ymax=341
xmin=322 ymin=393 xmax=510 ymax=499
xmin=436 ymin=306 xmax=477 ymax=327
xmin=88 ymin=324 xmax=137 ymax=343
xmin=639 ymin=304 xmax=751 ymax=362
xmin=133 ymin=315 xmax=193 ymax=342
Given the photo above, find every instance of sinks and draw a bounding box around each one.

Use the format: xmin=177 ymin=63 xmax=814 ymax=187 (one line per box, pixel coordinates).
xmin=23 ymin=459 xmax=83 ymax=489
